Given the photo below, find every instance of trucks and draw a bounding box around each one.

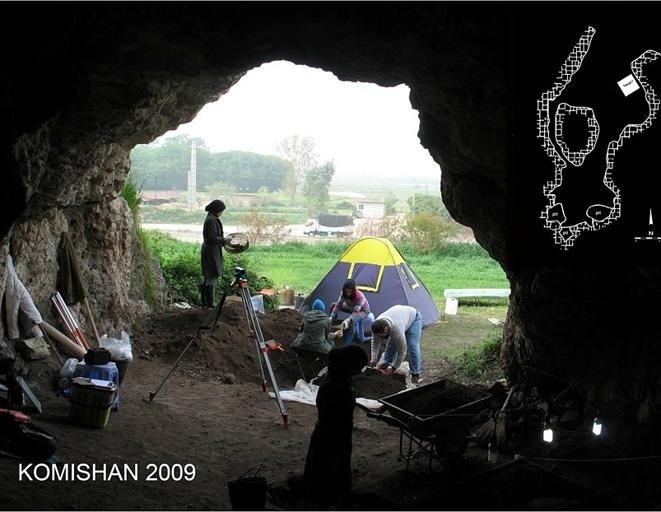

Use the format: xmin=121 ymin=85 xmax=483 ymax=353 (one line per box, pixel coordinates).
xmin=303 ymin=214 xmax=354 ymax=238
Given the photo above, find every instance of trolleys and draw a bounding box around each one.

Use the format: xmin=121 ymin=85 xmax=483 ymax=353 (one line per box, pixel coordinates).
xmin=364 ymin=379 xmax=493 ymax=475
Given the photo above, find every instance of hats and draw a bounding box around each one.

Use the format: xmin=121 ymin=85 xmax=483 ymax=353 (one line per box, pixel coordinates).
xmin=205 ymin=200 xmax=226 ymax=215
xmin=312 ymin=299 xmax=325 ymax=311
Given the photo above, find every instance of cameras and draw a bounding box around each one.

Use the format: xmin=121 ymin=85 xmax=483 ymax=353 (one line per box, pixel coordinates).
xmin=233 ymin=266 xmax=246 ymax=279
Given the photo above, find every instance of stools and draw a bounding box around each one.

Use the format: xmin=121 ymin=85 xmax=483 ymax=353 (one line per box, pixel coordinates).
xmin=72 ymin=360 xmax=122 ymax=413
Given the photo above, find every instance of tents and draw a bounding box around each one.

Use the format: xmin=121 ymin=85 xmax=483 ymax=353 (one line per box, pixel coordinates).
xmin=299 ymin=235 xmax=442 ymax=334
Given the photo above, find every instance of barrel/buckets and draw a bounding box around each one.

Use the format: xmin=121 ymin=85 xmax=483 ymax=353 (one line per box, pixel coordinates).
xmin=68 ymin=383 xmax=117 ymax=429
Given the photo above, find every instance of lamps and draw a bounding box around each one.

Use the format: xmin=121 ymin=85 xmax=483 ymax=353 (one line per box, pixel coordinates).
xmin=539 ymin=403 xmax=557 ymax=447
xmin=590 ymin=374 xmax=607 ymax=441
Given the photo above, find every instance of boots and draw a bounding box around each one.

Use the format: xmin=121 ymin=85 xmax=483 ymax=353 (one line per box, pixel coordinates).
xmin=205 ymin=284 xmax=215 ymax=310
xmin=199 ymin=284 xmax=205 ymax=309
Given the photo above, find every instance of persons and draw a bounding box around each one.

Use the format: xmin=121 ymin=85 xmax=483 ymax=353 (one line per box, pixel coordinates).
xmin=200 ymin=199 xmax=232 ymax=308
xmin=330 ymin=278 xmax=372 ymax=343
xmin=305 ymin=344 xmax=368 ymax=496
xmin=291 ymin=298 xmax=332 ymax=356
xmin=370 ymin=303 xmax=423 ymax=385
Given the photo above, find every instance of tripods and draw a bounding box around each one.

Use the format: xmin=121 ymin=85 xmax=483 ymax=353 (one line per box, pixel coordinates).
xmin=149 ymin=279 xmax=289 ymax=426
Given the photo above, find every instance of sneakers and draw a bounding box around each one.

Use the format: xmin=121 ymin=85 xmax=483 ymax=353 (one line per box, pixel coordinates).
xmin=411 ymin=373 xmax=419 ymax=382
